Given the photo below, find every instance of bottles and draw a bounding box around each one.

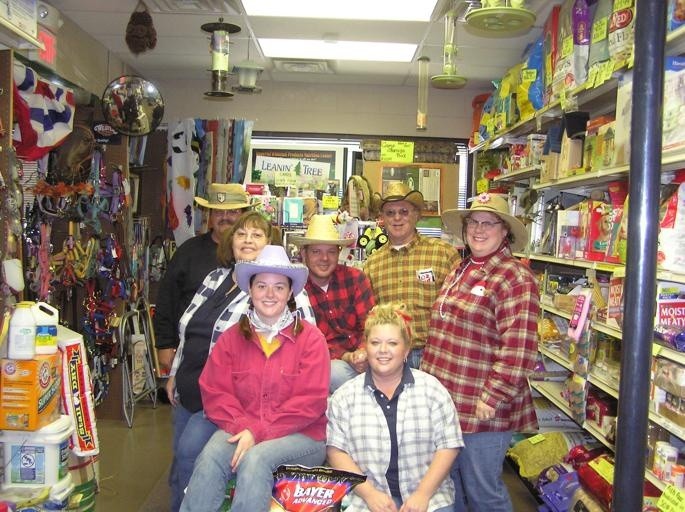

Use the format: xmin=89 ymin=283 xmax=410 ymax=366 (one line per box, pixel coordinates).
xmin=9 ymin=304 xmax=37 ymax=361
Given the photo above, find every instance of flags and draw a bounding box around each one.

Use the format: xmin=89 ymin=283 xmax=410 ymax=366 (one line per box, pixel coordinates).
xmin=16 ymin=60 xmax=76 ymax=162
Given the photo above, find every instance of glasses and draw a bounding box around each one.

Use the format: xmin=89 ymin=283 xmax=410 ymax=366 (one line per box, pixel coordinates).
xmin=466 ymin=218 xmax=501 ymax=228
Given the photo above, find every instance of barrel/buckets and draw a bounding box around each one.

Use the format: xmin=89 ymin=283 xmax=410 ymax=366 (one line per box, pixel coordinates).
xmin=21 ymin=301 xmax=59 ymax=355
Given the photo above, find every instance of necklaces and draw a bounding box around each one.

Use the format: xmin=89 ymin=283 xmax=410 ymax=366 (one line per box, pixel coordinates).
xmin=440 ymin=259 xmax=486 ymax=319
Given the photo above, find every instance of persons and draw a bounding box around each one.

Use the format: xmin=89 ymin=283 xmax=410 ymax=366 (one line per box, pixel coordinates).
xmin=325 ymin=305 xmax=466 ymax=512
xmin=289 ymin=215 xmax=378 ymax=394
xmin=365 ymin=181 xmax=464 ymax=371
xmin=418 ymin=191 xmax=539 ymax=512
xmin=156 ymin=184 xmax=260 ymax=371
xmin=164 ymin=213 xmax=283 ymax=512
xmin=180 ymin=243 xmax=331 ymax=512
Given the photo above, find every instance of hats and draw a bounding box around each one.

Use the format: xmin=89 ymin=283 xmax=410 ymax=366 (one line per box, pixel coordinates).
xmin=235 ymin=245 xmax=309 ymax=297
xmin=194 ymin=183 xmax=260 ymax=210
xmin=441 ymin=193 xmax=528 ymax=252
xmin=290 ymin=214 xmax=355 ymax=245
xmin=372 ymin=183 xmax=423 ymax=212
